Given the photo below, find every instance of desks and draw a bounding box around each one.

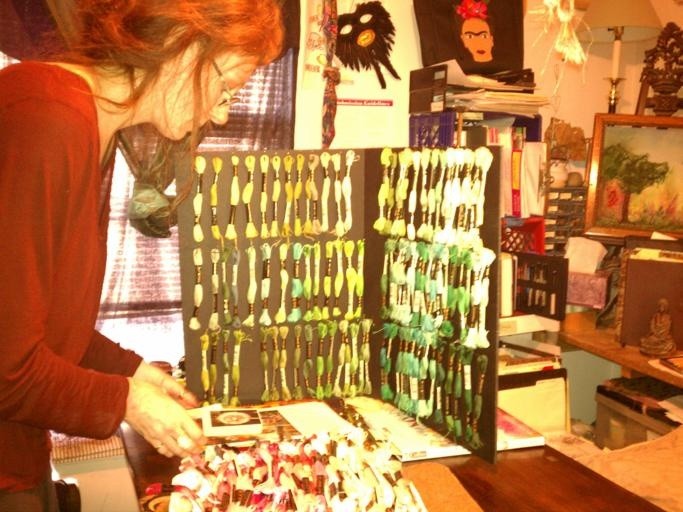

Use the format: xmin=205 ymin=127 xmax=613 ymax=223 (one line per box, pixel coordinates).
xmin=120 ymin=397 xmax=667 ymax=512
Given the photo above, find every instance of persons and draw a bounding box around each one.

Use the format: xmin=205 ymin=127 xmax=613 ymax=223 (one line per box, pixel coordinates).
xmin=0 ymin=0 xmax=286 ymax=512
xmin=640 ymin=298 xmax=674 ymax=355
xmin=460 ymin=16 xmax=495 ymax=63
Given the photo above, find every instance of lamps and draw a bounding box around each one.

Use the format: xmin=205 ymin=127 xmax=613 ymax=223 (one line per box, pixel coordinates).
xmin=579 ymin=0 xmax=662 ymax=114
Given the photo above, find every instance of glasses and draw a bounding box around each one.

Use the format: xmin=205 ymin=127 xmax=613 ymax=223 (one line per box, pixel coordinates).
xmin=202 ymin=35 xmax=243 ymax=110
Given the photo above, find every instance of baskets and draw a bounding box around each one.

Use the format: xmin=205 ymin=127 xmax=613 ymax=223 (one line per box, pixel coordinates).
xmin=498 ymin=216 xmax=547 ymax=318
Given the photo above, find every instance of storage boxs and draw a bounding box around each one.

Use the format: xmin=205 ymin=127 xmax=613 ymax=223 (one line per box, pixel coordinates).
xmin=585 ymin=376 xmax=681 ymax=453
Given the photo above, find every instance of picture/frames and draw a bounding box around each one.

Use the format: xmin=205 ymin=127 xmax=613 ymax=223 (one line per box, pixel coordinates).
xmin=583 ymin=109 xmax=682 ymax=239
